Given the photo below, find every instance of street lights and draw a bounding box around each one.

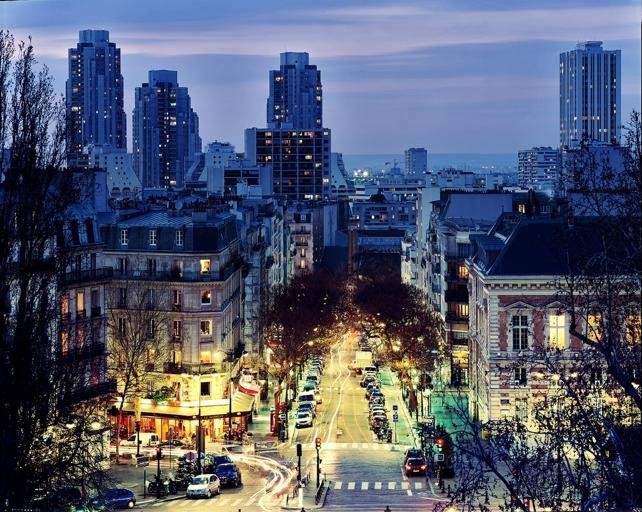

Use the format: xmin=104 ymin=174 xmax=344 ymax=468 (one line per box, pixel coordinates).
xmin=534 ymin=373 xmax=577 ymax=495
xmin=228 ymin=351 xmax=248 ymax=451
xmin=200 ymin=369 xmax=217 ymax=472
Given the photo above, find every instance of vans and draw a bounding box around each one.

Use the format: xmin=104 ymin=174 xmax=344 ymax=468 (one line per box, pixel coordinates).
xmin=120 ymin=432 xmax=158 ymax=446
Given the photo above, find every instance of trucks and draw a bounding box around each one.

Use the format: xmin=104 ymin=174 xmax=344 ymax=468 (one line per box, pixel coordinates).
xmin=349 ymin=359 xmax=372 ymax=373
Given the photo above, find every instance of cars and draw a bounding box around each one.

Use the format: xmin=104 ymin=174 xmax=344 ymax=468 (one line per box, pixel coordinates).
xmin=32 ymin=487 xmax=83 ymax=508
xmin=294 ymin=355 xmax=324 ymax=427
xmin=403 ymin=447 xmax=425 ymax=475
xmin=88 ymin=488 xmax=136 ymax=510
xmin=174 ymin=453 xmax=205 ymax=489
xmin=212 ymin=455 xmax=240 ymax=487
xmin=156 ymin=439 xmax=194 ymax=453
xmin=358 ymin=339 xmax=392 ymax=442
xmin=186 ymin=474 xmax=220 ymax=500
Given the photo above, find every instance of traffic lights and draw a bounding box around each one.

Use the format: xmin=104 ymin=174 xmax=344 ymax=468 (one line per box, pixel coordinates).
xmin=436 ymin=440 xmax=443 ymax=452
xmin=316 ymin=438 xmax=321 ymax=450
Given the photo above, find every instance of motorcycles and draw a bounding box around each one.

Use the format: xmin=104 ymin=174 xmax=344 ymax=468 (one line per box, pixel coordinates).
xmin=148 ymin=475 xmax=177 ymax=494
